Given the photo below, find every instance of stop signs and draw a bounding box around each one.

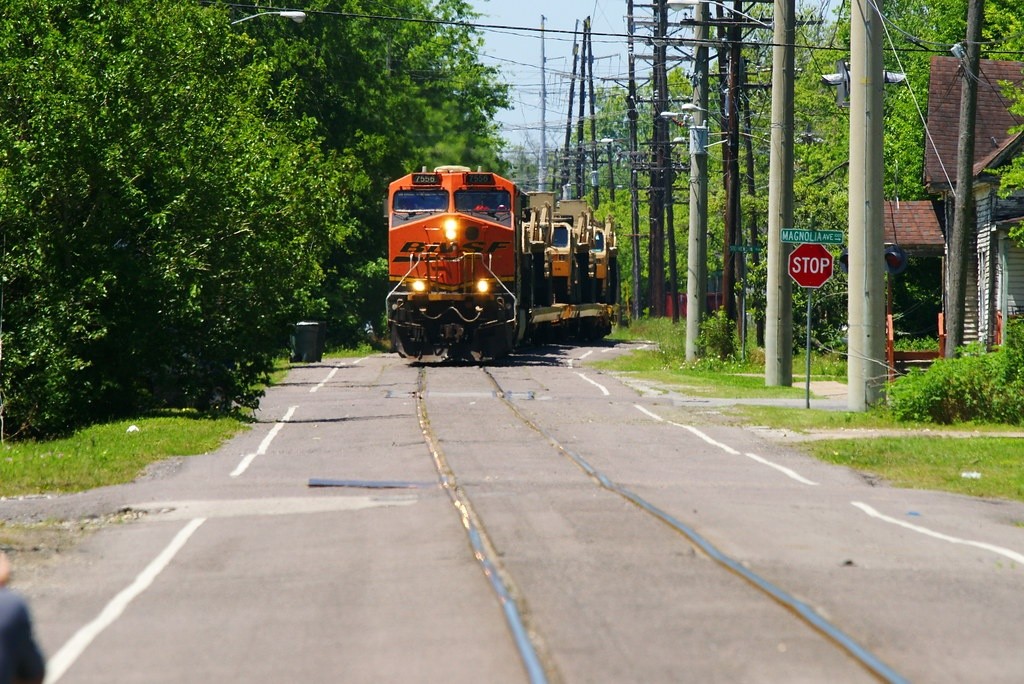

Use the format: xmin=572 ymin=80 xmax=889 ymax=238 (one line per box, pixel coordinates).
xmin=788 ymin=244 xmax=832 ymax=289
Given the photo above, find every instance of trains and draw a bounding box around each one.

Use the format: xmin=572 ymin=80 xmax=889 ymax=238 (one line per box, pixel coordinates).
xmin=385 ymin=164 xmax=622 ymax=360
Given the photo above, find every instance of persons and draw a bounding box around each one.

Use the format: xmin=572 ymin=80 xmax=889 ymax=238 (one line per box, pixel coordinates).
xmin=0 ymin=549 xmax=46 ymax=684
xmin=472 ymin=200 xmax=491 ymax=211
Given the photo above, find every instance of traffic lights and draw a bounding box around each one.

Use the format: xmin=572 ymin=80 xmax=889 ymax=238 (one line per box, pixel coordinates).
xmin=838 ymin=248 xmax=848 ymax=273
xmin=884 ymin=247 xmax=908 ymax=275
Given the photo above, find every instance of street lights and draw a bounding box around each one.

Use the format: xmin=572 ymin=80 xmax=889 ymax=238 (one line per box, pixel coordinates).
xmin=596 ymin=139 xmax=642 ymax=322
xmin=661 ymin=101 xmax=745 ymax=364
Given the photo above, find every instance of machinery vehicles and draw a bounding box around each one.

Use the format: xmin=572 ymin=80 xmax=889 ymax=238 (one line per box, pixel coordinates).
xmin=522 ymin=190 xmax=624 ymax=307
